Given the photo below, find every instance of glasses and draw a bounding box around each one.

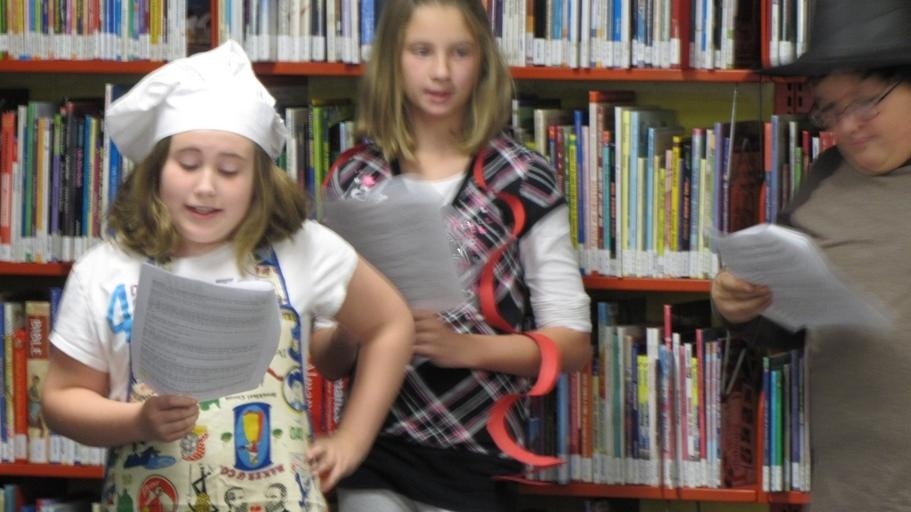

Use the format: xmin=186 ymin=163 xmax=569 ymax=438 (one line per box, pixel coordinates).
xmin=805 ymin=80 xmax=903 ymax=127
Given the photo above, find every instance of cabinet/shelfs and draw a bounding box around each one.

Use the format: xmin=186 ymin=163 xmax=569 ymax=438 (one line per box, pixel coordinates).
xmin=0 ymin=0 xmax=813 ymax=511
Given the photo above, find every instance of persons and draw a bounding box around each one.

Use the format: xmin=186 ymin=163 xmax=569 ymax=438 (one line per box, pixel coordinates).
xmin=711 ymin=0 xmax=911 ymax=512
xmin=321 ymin=2 xmax=591 ymax=510
xmin=41 ymin=40 xmax=415 ymax=511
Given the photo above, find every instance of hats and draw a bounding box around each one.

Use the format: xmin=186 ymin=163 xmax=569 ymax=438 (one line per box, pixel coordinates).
xmin=751 ymin=0 xmax=911 ymax=80
xmin=102 ymin=39 xmax=287 ymax=165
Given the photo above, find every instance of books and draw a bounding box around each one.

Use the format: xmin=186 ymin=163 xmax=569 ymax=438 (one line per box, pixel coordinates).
xmin=483 ymin=0 xmax=836 ymax=496
xmin=0 ymin=0 xmax=375 ymax=511
